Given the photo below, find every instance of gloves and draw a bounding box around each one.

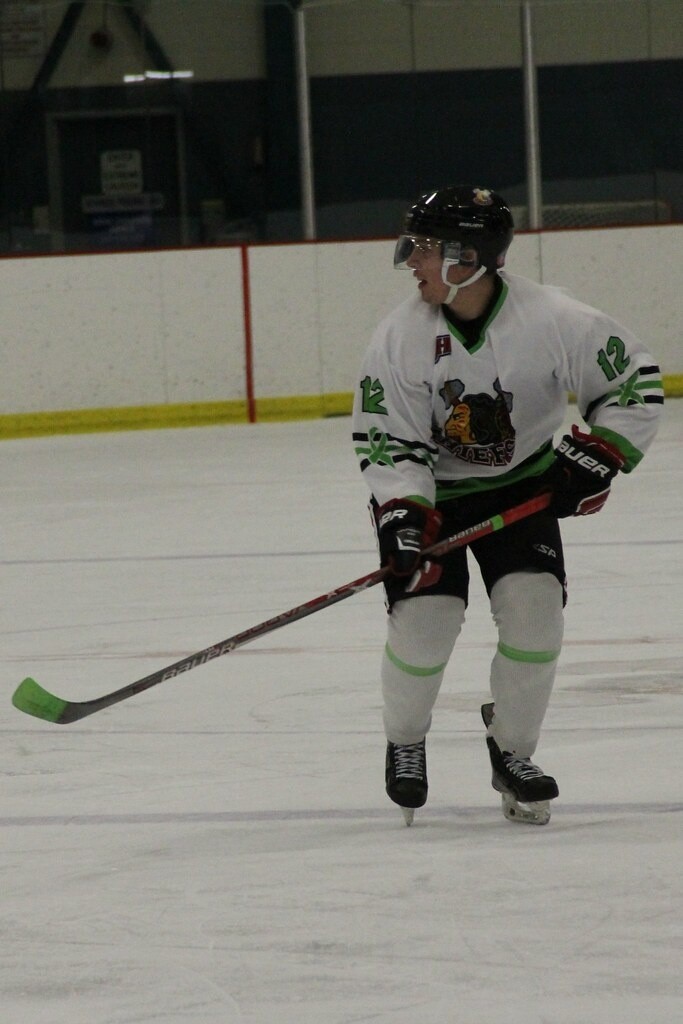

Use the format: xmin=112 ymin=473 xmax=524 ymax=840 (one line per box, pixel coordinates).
xmin=376 ymin=497 xmax=444 ymax=597
xmin=526 ymin=423 xmax=626 ymax=520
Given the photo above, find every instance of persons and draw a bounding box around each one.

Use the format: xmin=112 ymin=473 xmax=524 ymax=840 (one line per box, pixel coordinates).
xmin=348 ymin=174 xmax=668 ymax=833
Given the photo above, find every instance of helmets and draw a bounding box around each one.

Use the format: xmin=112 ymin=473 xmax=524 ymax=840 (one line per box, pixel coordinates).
xmin=394 ymin=186 xmax=515 ymax=288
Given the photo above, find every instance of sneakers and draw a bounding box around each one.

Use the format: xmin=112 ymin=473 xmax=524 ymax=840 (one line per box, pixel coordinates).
xmin=385 ymin=736 xmax=428 ymax=827
xmin=482 ymin=703 xmax=559 ymax=824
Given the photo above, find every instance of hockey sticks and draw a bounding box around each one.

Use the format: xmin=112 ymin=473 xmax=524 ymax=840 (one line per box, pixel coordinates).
xmin=8 ymin=487 xmax=558 ymax=727
